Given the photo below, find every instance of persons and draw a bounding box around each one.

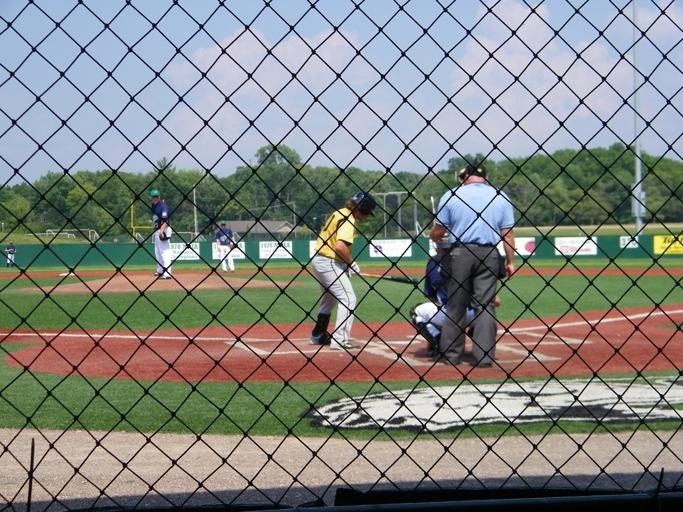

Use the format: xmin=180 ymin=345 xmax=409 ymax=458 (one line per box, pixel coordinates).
xmin=311 ymin=190 xmax=376 ymax=350
xmin=149 ymin=189 xmax=172 ymax=279
xmin=409 ymin=163 xmax=516 ymax=368
xmin=5 ymin=241 xmax=17 ymax=267
xmin=215 ymin=220 xmax=241 ymax=273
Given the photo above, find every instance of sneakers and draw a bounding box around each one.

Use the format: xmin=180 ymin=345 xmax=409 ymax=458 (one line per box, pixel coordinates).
xmin=445 ymin=355 xmax=493 ymax=367
xmin=153 ymin=272 xmax=172 ymax=280
xmin=308 ymin=332 xmax=331 ymax=345
xmin=329 ymin=338 xmax=361 ymax=350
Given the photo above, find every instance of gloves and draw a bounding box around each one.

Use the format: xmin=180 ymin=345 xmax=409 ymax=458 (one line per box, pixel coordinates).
xmin=348 ymin=261 xmax=360 ymax=278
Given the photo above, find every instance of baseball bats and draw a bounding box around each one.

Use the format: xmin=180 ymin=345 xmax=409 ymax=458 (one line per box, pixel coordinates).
xmin=346 ymin=270 xmax=418 ymax=284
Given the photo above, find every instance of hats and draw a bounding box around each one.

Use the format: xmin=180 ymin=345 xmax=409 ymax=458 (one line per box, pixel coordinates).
xmin=350 ymin=191 xmax=377 ymax=217
xmin=150 ymin=189 xmax=160 ymax=197
xmin=458 ymin=162 xmax=487 ymax=178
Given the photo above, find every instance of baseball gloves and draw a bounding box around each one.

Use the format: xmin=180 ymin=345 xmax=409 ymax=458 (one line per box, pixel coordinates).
xmin=160 ymin=232 xmax=171 ymax=240
